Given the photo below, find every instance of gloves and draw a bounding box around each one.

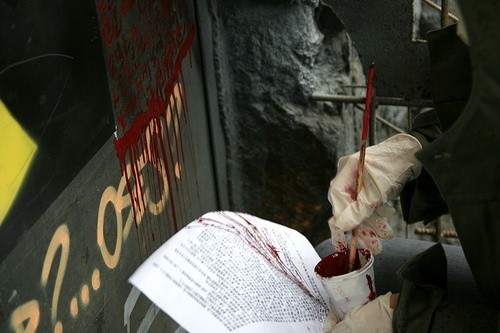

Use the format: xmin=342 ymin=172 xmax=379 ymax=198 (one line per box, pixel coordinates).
xmin=327 ymin=133 xmax=425 ymax=257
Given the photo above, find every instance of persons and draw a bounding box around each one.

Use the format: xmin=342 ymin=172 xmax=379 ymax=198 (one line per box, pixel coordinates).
xmin=314 ymin=0 xmax=500 ymax=333
